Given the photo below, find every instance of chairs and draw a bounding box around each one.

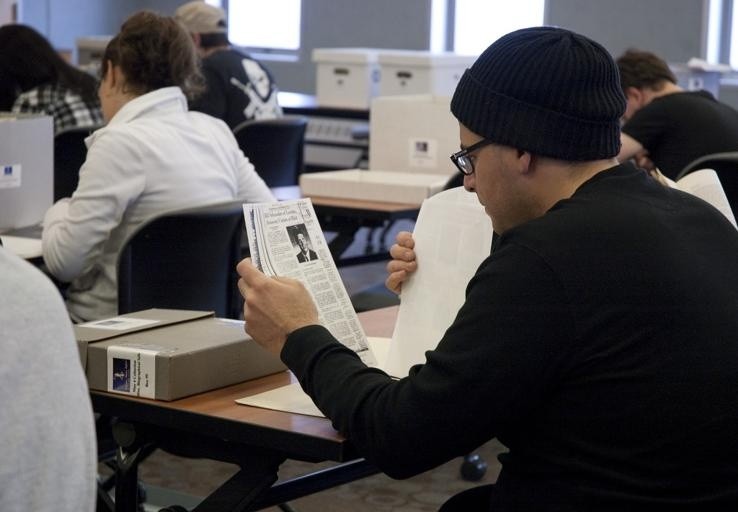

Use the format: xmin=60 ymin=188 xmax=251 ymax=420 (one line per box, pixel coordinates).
xmin=231 ymin=115 xmax=308 ymax=186
xmin=114 ymin=199 xmax=257 ymax=321
xmin=54 ymin=123 xmax=99 ymax=203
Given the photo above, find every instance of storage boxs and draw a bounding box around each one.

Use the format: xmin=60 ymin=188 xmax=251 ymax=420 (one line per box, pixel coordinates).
xmin=310 ymin=46 xmax=377 ymax=111
xmin=375 ymin=49 xmax=474 ymax=97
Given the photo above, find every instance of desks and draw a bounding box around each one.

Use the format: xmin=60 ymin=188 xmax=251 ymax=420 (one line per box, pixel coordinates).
xmin=90 ymin=300 xmax=496 ymax=511
xmin=302 ymin=194 xmax=420 ymax=267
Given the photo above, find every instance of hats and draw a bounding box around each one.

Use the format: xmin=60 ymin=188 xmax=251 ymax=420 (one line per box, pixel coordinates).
xmin=172 ymin=1 xmax=227 ymax=36
xmin=449 ymin=26 xmax=626 ymax=161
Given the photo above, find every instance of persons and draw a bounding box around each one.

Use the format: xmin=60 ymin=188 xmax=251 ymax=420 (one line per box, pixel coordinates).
xmin=614 ymin=46 xmax=737 ymax=225
xmin=235 ymin=25 xmax=737 ymax=512
xmin=292 ymin=227 xmax=317 ymax=263
xmin=0 ymin=23 xmax=105 ymax=135
xmin=40 ymin=9 xmax=277 ymax=322
xmin=175 ymin=2 xmax=283 ymax=133
xmin=0 ymin=245 xmax=100 ymax=512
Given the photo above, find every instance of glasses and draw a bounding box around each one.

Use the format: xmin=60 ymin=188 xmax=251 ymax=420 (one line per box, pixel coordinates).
xmin=448 ymin=139 xmax=486 ymax=176
